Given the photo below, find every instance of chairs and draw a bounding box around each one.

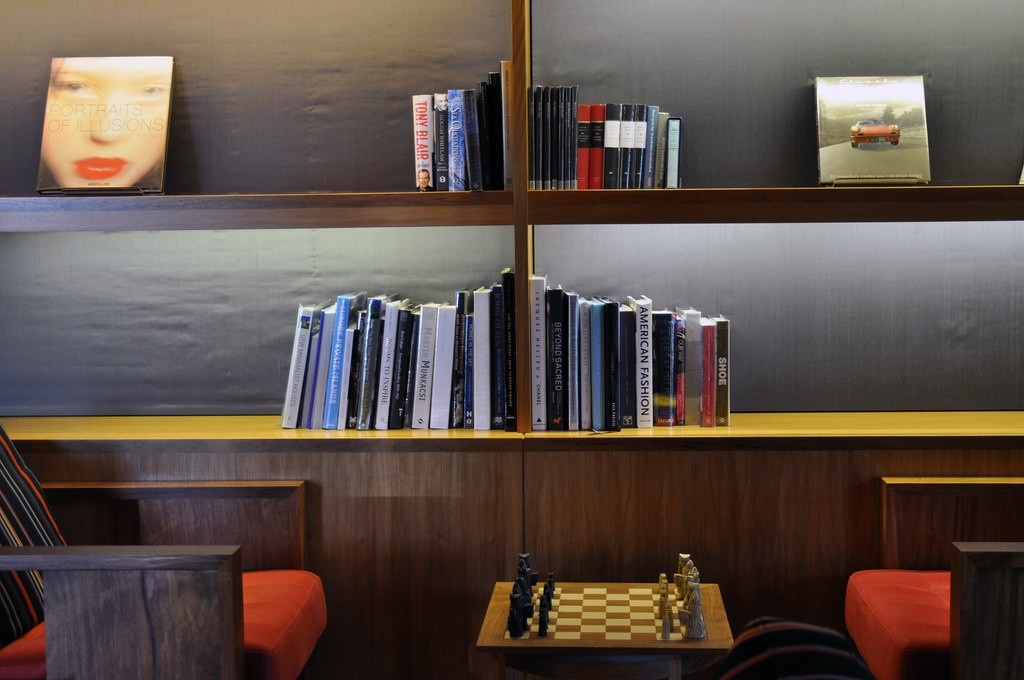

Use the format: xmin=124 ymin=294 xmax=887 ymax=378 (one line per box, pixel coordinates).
xmin=703 ymin=472 xmax=1022 ymax=679
xmin=0 ymin=425 xmax=327 ymax=680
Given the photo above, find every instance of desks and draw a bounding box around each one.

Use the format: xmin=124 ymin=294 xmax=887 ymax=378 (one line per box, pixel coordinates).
xmin=475 ymin=579 xmax=734 ymax=680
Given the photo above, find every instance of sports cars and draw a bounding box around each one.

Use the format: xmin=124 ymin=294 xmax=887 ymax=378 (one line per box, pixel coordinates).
xmin=850 ymin=118 xmax=901 ymax=148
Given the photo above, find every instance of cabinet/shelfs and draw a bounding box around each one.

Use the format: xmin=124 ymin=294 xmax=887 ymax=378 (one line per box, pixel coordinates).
xmin=0 ymin=1 xmax=1024 ymax=680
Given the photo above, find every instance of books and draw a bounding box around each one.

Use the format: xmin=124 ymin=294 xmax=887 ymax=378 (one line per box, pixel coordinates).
xmin=35 ymin=56 xmax=174 ymax=192
xmin=530 ymin=273 xmax=731 ymax=432
xmin=814 ymin=75 xmax=931 ymax=185
xmin=412 ymin=61 xmax=515 ymax=192
xmin=282 ymin=268 xmax=518 ymax=432
xmin=527 ymin=84 xmax=683 ymax=191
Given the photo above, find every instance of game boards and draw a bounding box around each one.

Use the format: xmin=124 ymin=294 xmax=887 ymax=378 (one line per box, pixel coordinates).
xmin=504 ymin=587 xmax=686 ymax=640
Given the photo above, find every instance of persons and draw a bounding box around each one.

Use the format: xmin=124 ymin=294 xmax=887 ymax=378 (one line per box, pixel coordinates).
xmin=40 ymin=57 xmax=173 ymax=187
xmin=416 ymin=169 xmax=433 ymax=192
